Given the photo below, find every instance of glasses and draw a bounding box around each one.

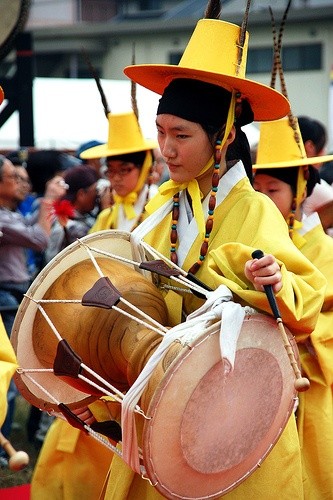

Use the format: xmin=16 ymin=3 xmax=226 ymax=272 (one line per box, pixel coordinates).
xmin=104 ymin=166 xmax=136 ymax=178
xmin=7 ymin=173 xmax=20 ymax=182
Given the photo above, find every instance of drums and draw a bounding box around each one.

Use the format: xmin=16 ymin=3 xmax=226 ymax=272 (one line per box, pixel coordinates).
xmin=10 ymin=229 xmax=300 ymax=500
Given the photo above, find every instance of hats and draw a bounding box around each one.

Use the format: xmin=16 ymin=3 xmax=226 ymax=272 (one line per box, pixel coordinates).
xmin=75 ymin=140 xmax=105 ymax=156
xmin=251 ymin=0 xmax=333 ymax=199
xmin=79 ymin=38 xmax=159 ymax=160
xmin=123 ymin=0 xmax=291 ymax=155
xmin=60 ymin=168 xmax=101 ymax=199
xmin=30 ymin=152 xmax=63 ymax=192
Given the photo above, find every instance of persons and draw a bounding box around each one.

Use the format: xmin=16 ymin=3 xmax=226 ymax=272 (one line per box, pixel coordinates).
xmin=0 ymin=140 xmax=117 ymax=470
xmin=71 ymin=16 xmax=327 ymax=500
xmin=27 ymin=112 xmax=333 ymax=500
